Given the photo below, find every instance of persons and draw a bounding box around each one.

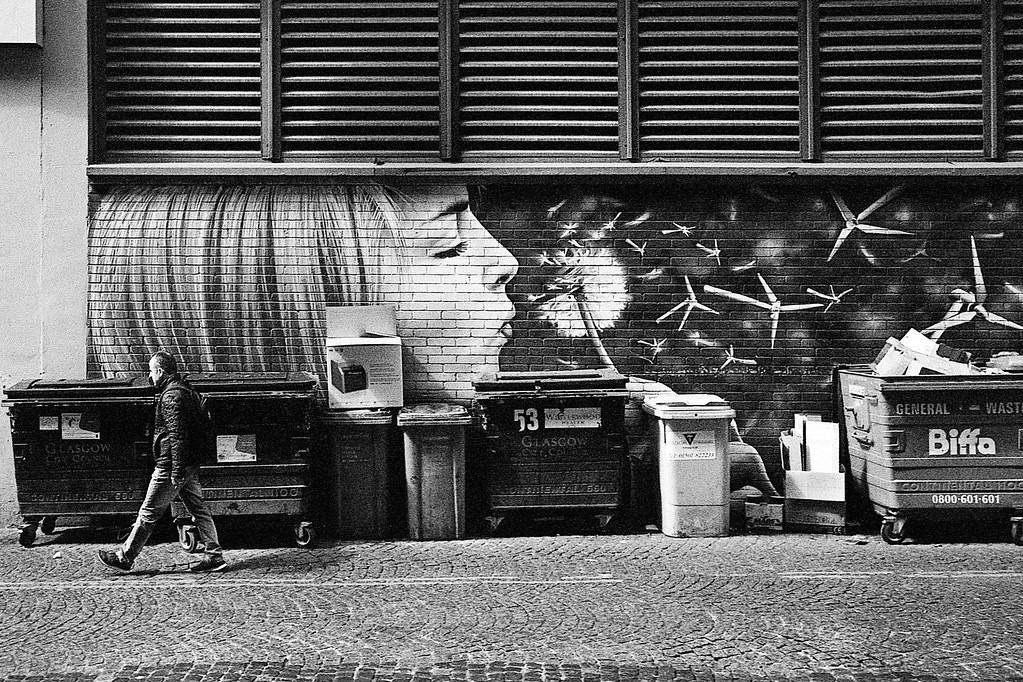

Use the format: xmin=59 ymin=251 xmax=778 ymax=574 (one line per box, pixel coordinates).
xmin=91 ymin=179 xmax=516 ymax=408
xmin=97 ymin=352 xmax=227 ymax=574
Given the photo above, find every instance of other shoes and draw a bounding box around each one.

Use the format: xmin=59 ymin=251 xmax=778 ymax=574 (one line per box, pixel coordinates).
xmin=190 ymin=555 xmax=227 ymax=573
xmin=97 ymin=550 xmax=130 ymax=574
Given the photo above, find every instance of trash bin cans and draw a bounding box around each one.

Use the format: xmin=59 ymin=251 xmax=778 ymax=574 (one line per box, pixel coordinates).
xmin=322 ymin=409 xmax=396 ymax=543
xmin=838 ymin=368 xmax=1022 ymax=545
xmin=398 ymin=402 xmax=477 ymax=541
xmin=640 ymin=391 xmax=738 ymax=539
xmin=471 ymin=366 xmax=634 ymax=535
xmin=1 ymin=371 xmax=320 ymax=548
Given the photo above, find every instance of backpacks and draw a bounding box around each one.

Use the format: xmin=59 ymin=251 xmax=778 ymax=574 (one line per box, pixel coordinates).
xmin=158 ymin=386 xmax=215 ymax=466
